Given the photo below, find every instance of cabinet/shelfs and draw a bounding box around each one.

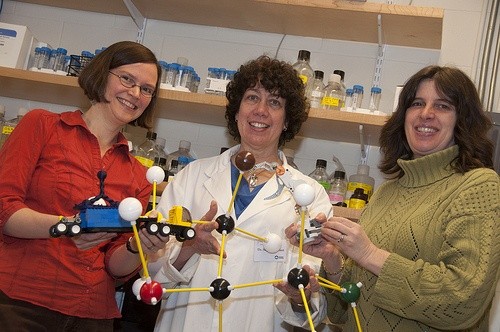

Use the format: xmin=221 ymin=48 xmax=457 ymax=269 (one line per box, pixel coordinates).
xmin=0 ymin=0 xmax=445 ymax=221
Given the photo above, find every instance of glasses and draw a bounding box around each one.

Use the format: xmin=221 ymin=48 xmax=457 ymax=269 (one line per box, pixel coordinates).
xmin=108 ymin=70 xmax=156 ymax=97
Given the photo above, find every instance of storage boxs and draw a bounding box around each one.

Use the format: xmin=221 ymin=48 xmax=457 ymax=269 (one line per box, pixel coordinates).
xmin=0 ymin=22 xmax=39 ymax=70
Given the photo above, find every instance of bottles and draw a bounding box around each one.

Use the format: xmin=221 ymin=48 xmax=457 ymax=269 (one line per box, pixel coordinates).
xmin=282 ymin=148 xmax=376 ymax=210
xmin=130 ymin=132 xmax=195 ymax=177
xmin=292 ymin=48 xmax=347 ymax=112
xmin=0 ymin=104 xmax=29 ymax=151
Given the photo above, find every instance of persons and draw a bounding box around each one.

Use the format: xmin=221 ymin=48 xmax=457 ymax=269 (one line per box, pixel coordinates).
xmin=285 ymin=66 xmax=500 ymax=332
xmin=138 ymin=54 xmax=334 ymax=332
xmin=0 ymin=40 xmax=169 ymax=332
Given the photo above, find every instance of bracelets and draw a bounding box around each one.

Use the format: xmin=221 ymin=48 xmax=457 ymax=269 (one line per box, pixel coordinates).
xmin=293 ymin=298 xmax=311 ymax=307
xmin=323 ymin=257 xmax=344 ymax=275
xmin=127 ymin=236 xmax=139 ymax=254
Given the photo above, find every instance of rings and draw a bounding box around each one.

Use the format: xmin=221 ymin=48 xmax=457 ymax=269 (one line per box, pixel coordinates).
xmin=339 ymin=233 xmax=344 ymax=241
